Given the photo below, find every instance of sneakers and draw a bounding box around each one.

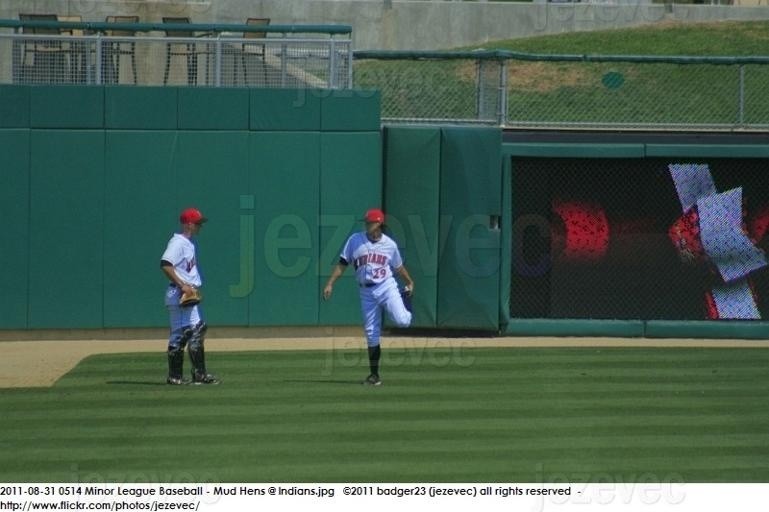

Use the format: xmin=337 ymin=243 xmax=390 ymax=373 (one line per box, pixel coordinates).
xmin=401 ymin=282 xmax=414 ymax=293
xmin=362 ymin=374 xmax=382 ymax=385
xmin=167 ymin=374 xmax=223 ymax=385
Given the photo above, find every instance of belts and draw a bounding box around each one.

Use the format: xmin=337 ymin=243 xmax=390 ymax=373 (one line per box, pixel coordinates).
xmin=170 ymin=284 xmax=176 ymax=288
xmin=360 ymin=283 xmax=376 ymax=287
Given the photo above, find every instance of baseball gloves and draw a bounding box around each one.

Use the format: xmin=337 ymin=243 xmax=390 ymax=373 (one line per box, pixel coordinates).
xmin=179 ymin=287 xmax=201 ymax=306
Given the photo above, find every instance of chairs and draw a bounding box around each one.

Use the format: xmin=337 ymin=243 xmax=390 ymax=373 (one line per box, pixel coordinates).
xmin=161 ymin=16 xmax=270 ymax=87
xmin=16 ymin=14 xmax=140 ymax=86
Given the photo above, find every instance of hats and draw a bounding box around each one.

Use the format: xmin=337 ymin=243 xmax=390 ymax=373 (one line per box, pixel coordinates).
xmin=180 ymin=208 xmax=208 ymax=223
xmin=364 ymin=208 xmax=384 ymax=222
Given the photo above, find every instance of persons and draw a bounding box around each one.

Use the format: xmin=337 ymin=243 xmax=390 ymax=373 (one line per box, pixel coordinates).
xmin=160 ymin=208 xmax=221 ymax=386
xmin=322 ymin=209 xmax=414 ymax=385
